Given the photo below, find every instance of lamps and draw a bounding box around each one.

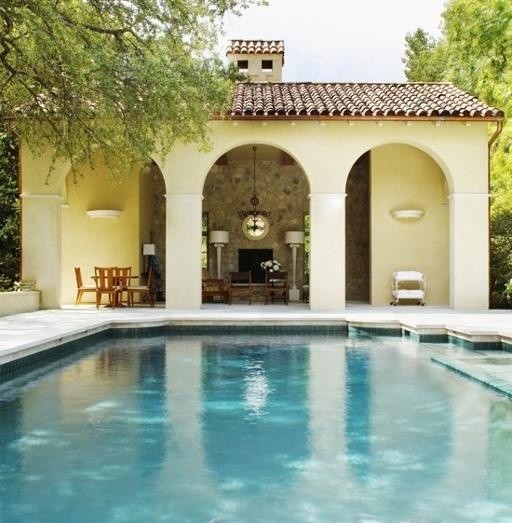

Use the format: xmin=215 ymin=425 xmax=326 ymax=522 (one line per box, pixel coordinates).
xmin=210 ymin=230 xmax=229 ymax=278
xmin=143 ymin=244 xmax=155 ymax=269
xmin=284 ymin=231 xmax=304 ymax=300
xmin=238 ymin=146 xmax=274 ymax=223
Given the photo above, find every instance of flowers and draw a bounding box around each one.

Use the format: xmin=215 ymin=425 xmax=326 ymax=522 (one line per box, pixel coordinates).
xmin=261 ymin=259 xmax=282 ymax=271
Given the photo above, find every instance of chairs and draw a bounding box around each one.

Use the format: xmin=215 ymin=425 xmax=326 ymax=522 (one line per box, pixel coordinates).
xmin=75 ymin=266 xmax=155 ymax=308
xmin=201 ymin=268 xmax=290 ymax=305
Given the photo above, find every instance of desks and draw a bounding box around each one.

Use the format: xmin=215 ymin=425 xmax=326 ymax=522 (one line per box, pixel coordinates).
xmin=391 ymin=275 xmax=428 ymax=306
